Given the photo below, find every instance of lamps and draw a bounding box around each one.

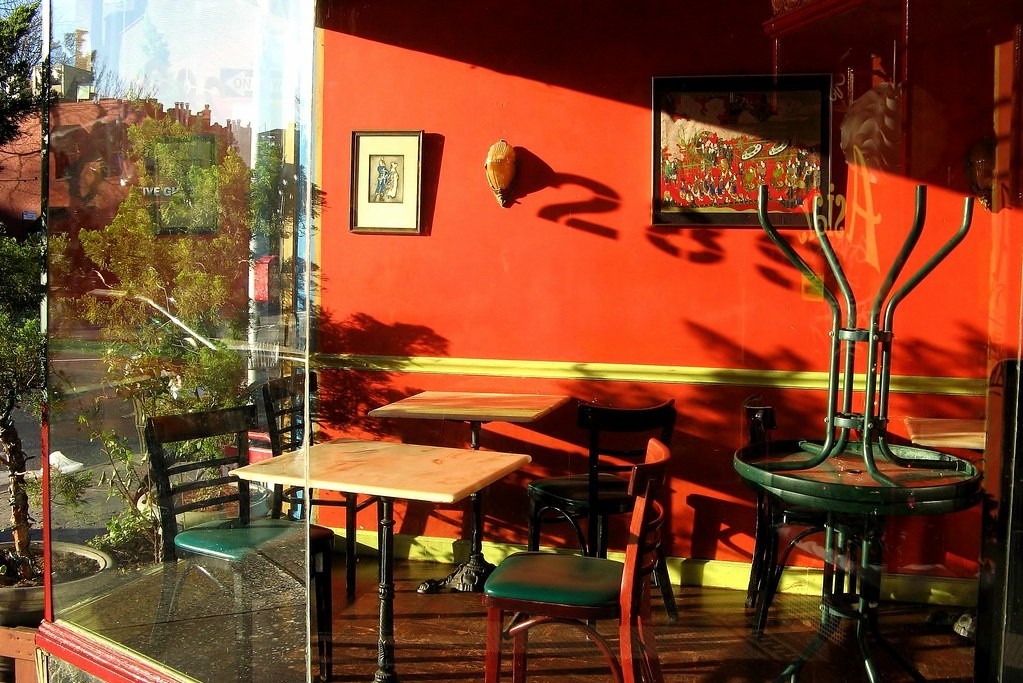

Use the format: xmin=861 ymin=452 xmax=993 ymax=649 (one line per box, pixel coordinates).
xmin=483 ymin=139 xmax=517 ymax=207
xmin=967 ymin=136 xmax=998 ymax=211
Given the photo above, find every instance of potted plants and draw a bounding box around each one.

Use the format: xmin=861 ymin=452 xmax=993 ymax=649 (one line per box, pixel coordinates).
xmin=50 ymin=101 xmax=285 ymax=530
xmin=0 ymin=223 xmax=118 ymax=674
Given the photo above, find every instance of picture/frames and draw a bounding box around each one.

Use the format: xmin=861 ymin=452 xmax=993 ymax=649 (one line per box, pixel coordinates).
xmin=651 ymin=71 xmax=834 ymax=230
xmin=348 ymin=130 xmax=424 ymax=235
xmin=146 ymin=134 xmax=221 ymax=237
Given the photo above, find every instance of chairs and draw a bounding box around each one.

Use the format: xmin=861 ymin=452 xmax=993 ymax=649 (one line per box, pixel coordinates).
xmin=258 ymin=372 xmax=384 ymax=602
xmin=480 ymin=437 xmax=673 ymax=683
xmin=501 ymin=396 xmax=677 ymax=641
xmin=742 ymin=404 xmax=862 ymax=608
xmin=144 ymin=405 xmax=333 ymax=683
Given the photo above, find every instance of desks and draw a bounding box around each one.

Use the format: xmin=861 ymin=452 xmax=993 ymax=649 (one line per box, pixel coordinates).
xmin=367 ymin=392 xmax=571 ymax=595
xmin=228 ymin=439 xmax=533 ymax=682
xmin=732 ymin=184 xmax=983 ymax=682
